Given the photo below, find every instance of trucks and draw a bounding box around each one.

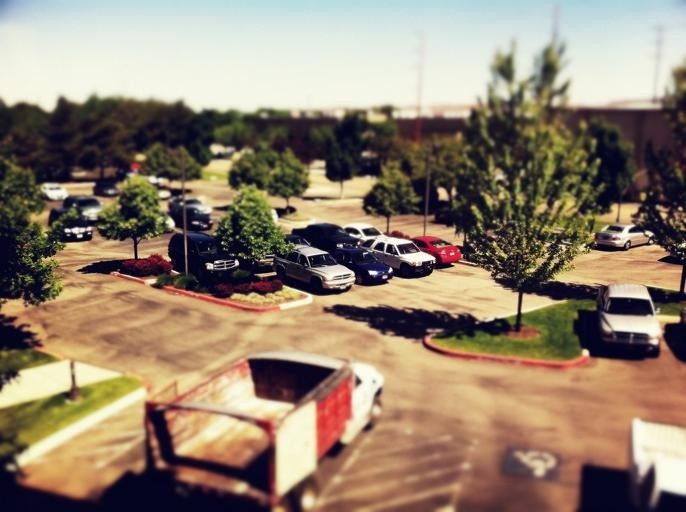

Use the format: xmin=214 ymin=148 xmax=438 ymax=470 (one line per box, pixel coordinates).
xmin=145 ymin=351 xmax=383 ymax=512
xmin=630 ymin=417 xmax=686 ymax=512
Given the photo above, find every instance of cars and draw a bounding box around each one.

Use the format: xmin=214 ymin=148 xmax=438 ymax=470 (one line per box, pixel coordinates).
xmin=597 ymin=283 xmax=661 ymax=356
xmin=94 ymin=186 xmax=118 ymax=196
xmin=48 ymin=195 xmax=103 ymax=240
xmin=248 ymin=223 xmax=462 ymax=292
xmin=595 ymin=224 xmax=656 ymax=250
xmin=168 ymin=231 xmax=240 ymax=274
xmin=41 ymin=183 xmax=69 ymax=201
xmin=169 ymin=195 xmax=213 ymax=229
